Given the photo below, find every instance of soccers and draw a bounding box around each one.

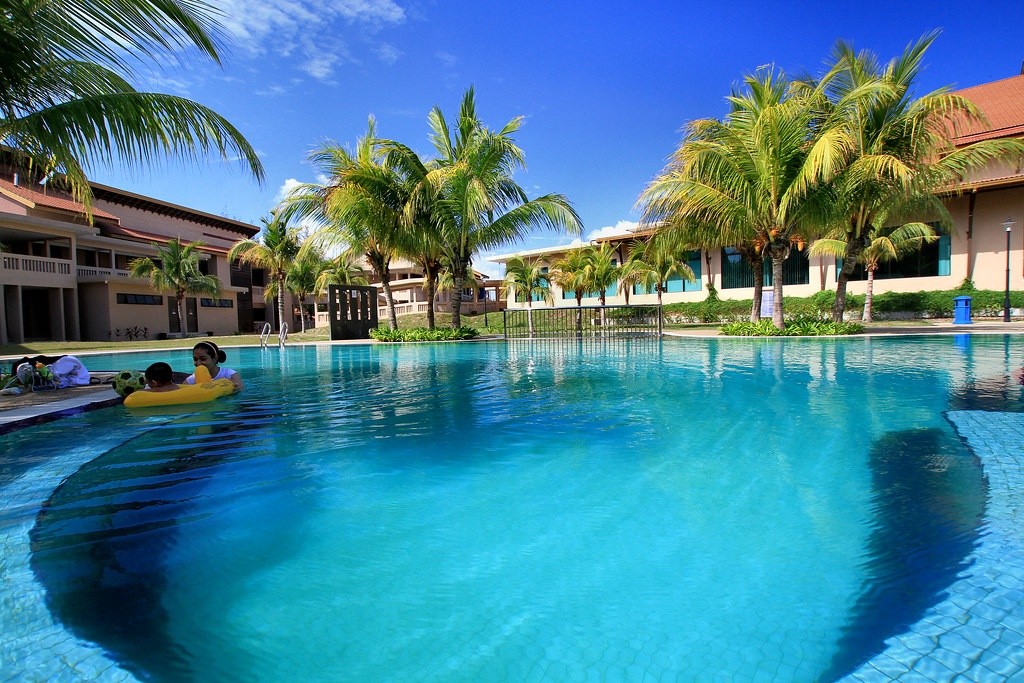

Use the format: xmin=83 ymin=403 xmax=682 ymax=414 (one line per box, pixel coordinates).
xmin=112 ymin=370 xmax=146 ymax=397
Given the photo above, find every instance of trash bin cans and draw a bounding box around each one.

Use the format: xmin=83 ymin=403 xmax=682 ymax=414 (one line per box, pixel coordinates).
xmin=952 ymin=295 xmax=973 ymax=324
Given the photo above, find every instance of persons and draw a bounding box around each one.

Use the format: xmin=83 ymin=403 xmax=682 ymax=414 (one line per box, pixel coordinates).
xmin=144 ymin=361 xmax=181 ymax=393
xmin=181 ymin=340 xmax=243 ymax=390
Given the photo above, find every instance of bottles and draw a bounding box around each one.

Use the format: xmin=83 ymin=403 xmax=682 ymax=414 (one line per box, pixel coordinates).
xmin=36 ymin=363 xmax=53 ymax=380
